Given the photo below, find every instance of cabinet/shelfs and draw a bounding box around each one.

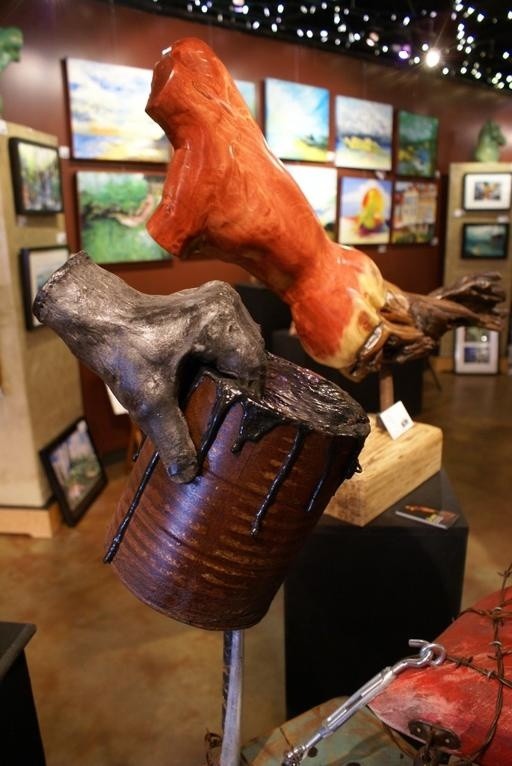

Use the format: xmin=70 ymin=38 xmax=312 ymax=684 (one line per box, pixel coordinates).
xmin=284 ymin=470 xmax=467 ymax=720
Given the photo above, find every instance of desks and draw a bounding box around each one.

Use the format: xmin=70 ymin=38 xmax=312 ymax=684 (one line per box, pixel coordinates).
xmin=0 ymin=620 xmax=46 ymax=765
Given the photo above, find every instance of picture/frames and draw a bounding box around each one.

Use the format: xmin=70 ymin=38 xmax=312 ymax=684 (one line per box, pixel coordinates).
xmin=462 ymin=172 xmax=511 ymax=211
xmin=462 ymin=222 xmax=509 ymax=260
xmin=8 ymin=135 xmax=65 ymax=216
xmin=454 ymin=309 xmax=500 ymax=377
xmin=21 ymin=244 xmax=70 ymax=330
xmin=37 ymin=417 xmax=108 ymax=526
